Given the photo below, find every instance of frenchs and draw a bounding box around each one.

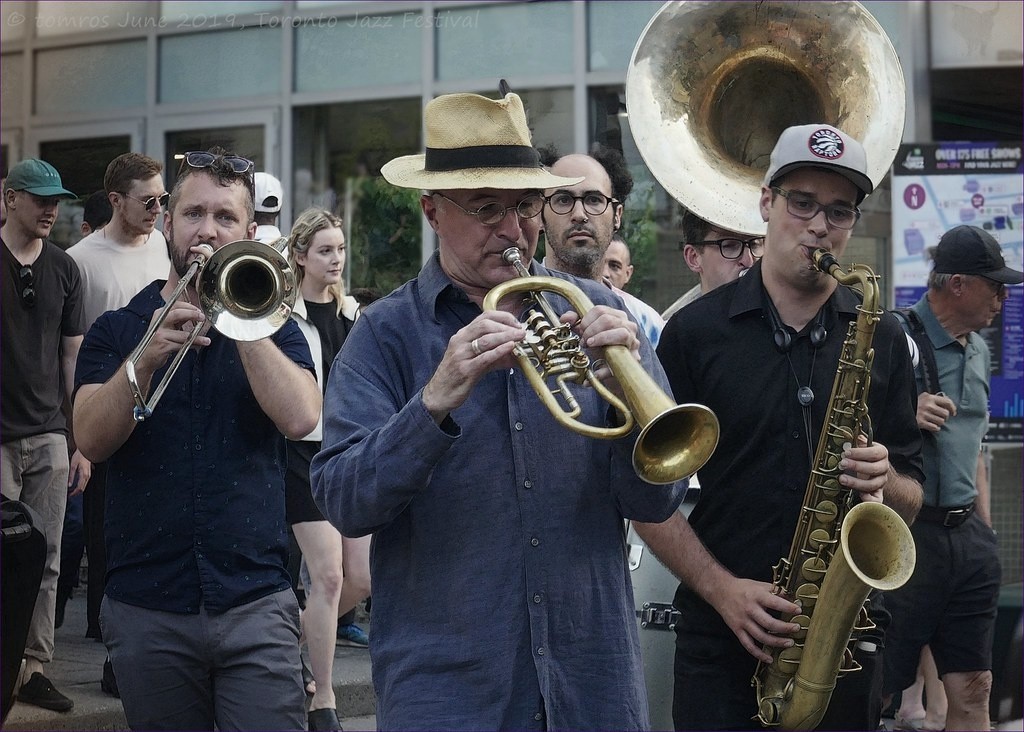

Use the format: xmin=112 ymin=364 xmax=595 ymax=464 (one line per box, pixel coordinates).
xmin=625 ymin=0 xmax=906 ymax=322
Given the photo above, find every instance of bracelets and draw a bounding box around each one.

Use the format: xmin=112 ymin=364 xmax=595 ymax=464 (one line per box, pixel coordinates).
xmin=992 ymin=528 xmax=999 ymax=537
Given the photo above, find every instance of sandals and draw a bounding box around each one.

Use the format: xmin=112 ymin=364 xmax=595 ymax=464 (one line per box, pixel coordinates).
xmin=893 ymin=713 xmax=925 ymax=730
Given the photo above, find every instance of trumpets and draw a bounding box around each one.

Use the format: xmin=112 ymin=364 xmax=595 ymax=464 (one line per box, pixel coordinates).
xmin=481 ymin=244 xmax=721 ymax=486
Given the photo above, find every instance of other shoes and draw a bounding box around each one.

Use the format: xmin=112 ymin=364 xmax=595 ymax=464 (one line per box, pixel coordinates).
xmin=85 ymin=614 xmax=103 ymax=642
xmin=101 ymin=655 xmax=120 ymax=699
xmin=879 ymin=724 xmax=887 ymax=732
xmin=881 ymin=704 xmax=896 ymax=720
xmin=308 ymin=708 xmax=343 ymax=732
xmin=300 ymin=655 xmax=316 ymax=697
xmin=54 ymin=585 xmax=73 ymax=629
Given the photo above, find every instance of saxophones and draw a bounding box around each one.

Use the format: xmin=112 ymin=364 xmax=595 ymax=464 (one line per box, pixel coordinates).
xmin=752 ymin=253 xmax=917 ymax=732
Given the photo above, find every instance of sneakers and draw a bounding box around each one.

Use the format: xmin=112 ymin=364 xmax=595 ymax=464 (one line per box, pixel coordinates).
xmin=17 ymin=672 xmax=74 ymax=711
xmin=336 ymin=625 xmax=369 ymax=648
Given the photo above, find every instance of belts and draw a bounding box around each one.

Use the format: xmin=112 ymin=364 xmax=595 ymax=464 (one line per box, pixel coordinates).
xmin=916 ymin=501 xmax=977 ymax=526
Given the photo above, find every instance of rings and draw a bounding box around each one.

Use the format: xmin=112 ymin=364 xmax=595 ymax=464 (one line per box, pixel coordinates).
xmin=471 ymin=339 xmax=481 ymax=355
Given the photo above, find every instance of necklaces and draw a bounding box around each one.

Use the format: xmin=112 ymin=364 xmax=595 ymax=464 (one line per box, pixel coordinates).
xmin=1 ymin=227 xmax=39 ymax=262
xmin=103 ymin=225 xmax=150 ymax=243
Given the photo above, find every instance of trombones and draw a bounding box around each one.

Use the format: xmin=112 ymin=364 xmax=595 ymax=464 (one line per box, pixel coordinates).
xmin=124 ymin=244 xmax=299 ymax=422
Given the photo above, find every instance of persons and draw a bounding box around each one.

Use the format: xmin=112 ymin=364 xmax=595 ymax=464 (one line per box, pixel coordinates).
xmin=71 ymin=146 xmax=323 ymax=732
xmin=539 ymin=143 xmax=667 ymax=352
xmin=0 ymin=157 xmax=384 ymax=732
xmin=310 ymin=92 xmax=690 ymax=732
xmin=602 ymin=232 xmax=634 ymax=290
xmin=878 ymin=226 xmax=1024 ymax=732
xmin=630 ymin=123 xmax=926 ymax=732
xmin=660 ymin=211 xmax=919 ymax=368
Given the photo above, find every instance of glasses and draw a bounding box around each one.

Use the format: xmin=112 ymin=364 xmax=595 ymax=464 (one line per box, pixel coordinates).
xmin=20 ymin=264 xmax=37 ymax=307
xmin=174 ymin=151 xmax=255 ymax=184
xmin=431 ymin=191 xmax=548 ymax=225
xmin=541 ymin=192 xmax=619 ymax=215
xmin=126 ymin=191 xmax=169 ymax=211
xmin=682 ymin=237 xmax=766 ymax=259
xmin=772 ymin=188 xmax=861 ymax=230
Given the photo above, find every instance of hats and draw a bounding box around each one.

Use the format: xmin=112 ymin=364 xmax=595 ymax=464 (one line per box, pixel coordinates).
xmin=246 ymin=172 xmax=282 ymax=212
xmin=936 ymin=225 xmax=1024 ymax=284
xmin=381 ymin=92 xmax=584 ymax=189
xmin=764 ymin=124 xmax=874 ymax=195
xmin=3 ymin=158 xmax=78 ymax=198
